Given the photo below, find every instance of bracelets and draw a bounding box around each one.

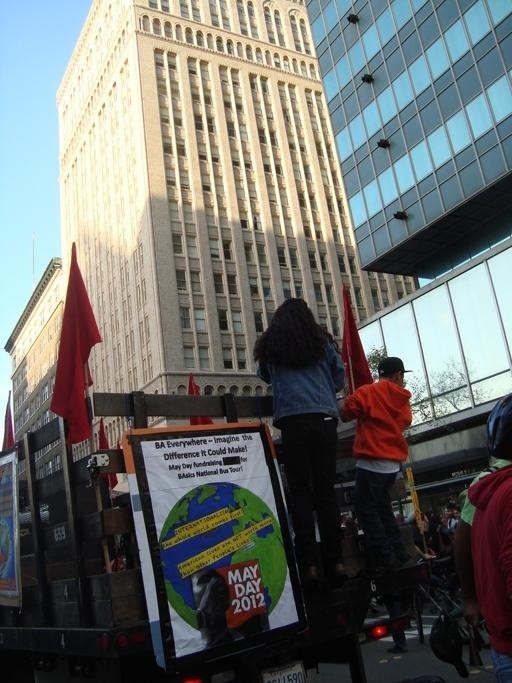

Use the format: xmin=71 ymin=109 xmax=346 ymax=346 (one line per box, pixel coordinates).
xmin=198 ymin=608 xmax=208 ymax=626
xmin=334 ymin=395 xmax=344 ymax=401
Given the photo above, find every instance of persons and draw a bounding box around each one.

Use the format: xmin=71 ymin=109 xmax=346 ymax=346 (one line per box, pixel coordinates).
xmin=254 ymin=296 xmax=347 ymax=587
xmin=341 ymin=391 xmax=512 ymax=683
xmin=335 ymin=356 xmax=414 ymax=574
xmin=191 ymin=570 xmax=263 ymax=651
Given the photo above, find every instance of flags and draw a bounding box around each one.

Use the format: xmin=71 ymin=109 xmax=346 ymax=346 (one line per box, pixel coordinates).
xmin=49 ymin=244 xmax=102 ymax=451
xmin=186 ymin=372 xmax=218 ymax=426
xmin=98 ymin=416 xmax=118 ymax=491
xmin=2 ymin=402 xmax=16 ymax=451
xmin=339 ymin=284 xmax=373 ymax=395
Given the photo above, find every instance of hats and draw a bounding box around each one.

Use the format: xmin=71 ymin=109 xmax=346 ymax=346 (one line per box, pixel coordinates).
xmin=429 ymin=613 xmax=470 ymax=678
xmin=378 ymin=356 xmax=413 ymax=375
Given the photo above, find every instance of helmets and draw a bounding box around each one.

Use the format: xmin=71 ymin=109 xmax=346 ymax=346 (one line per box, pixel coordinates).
xmin=487 ymin=392 xmax=512 ymax=456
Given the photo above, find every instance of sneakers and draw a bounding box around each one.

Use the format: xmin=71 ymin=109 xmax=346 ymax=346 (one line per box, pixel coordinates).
xmin=387 ymin=643 xmax=409 ymax=653
